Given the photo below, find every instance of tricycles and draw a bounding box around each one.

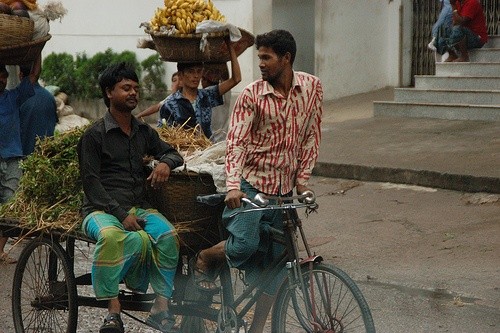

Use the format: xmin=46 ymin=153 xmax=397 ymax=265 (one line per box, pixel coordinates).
xmin=0 ymin=189 xmax=376 ymax=333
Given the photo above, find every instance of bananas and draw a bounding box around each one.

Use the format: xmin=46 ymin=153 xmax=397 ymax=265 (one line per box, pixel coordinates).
xmin=150 ymin=0 xmax=226 ymax=34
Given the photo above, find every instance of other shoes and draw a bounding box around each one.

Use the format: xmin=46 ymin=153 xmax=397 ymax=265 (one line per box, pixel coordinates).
xmin=452 ymin=57 xmax=470 ymax=62
xmin=444 ymin=56 xmax=457 ymax=62
xmin=1 ymin=254 xmax=17 ymax=265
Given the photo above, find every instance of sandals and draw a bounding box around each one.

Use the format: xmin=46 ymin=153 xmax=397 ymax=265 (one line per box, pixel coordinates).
xmin=188 ymin=250 xmax=218 ymax=293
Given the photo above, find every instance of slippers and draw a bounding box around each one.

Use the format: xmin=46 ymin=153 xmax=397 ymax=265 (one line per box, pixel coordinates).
xmin=99 ymin=312 xmax=125 ymax=332
xmin=146 ymin=309 xmax=181 ymax=333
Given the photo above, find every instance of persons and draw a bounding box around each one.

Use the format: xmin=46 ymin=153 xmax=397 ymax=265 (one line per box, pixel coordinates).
xmin=0 ymin=51 xmax=41 ymax=261
xmin=77 ymin=61 xmax=184 ymax=333
xmin=434 ymin=0 xmax=488 ymax=62
xmin=134 ymin=72 xmax=183 ymax=119
xmin=159 ymin=36 xmax=243 ymax=143
xmin=18 ymin=64 xmax=58 ymax=159
xmin=188 ymin=29 xmax=324 ymax=333
